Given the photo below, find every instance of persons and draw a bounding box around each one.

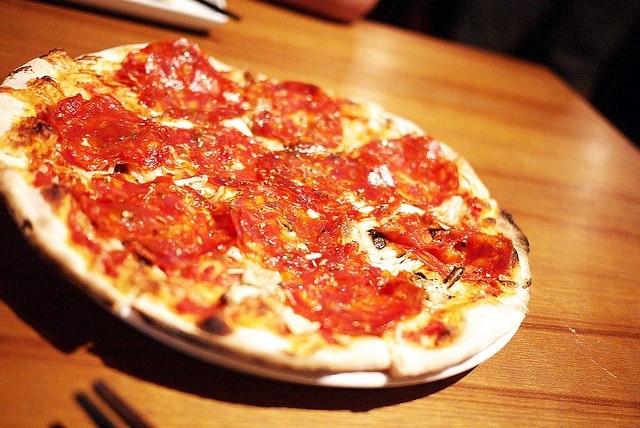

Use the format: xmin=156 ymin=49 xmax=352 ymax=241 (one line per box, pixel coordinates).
xmin=276 ymin=0 xmax=379 ymax=24
